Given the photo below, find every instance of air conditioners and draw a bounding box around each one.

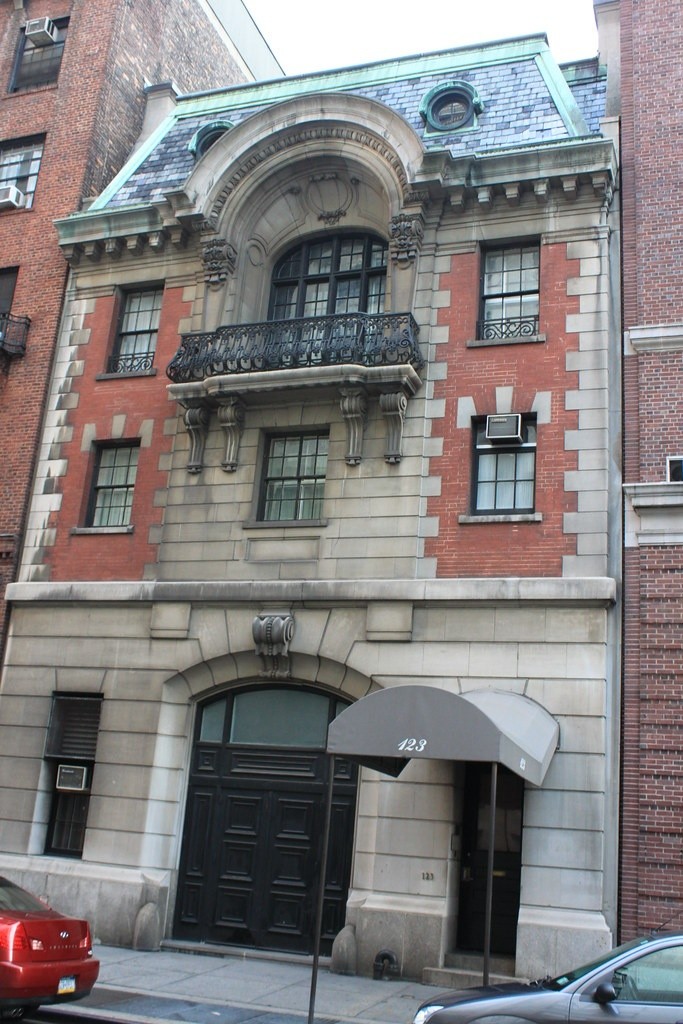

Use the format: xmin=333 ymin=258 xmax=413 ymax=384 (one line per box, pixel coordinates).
xmin=0 ymin=186 xmax=26 ymax=210
xmin=55 ymin=763 xmax=87 ymax=792
xmin=24 ymin=16 xmax=59 ymax=47
xmin=485 ymin=413 xmax=530 ymax=445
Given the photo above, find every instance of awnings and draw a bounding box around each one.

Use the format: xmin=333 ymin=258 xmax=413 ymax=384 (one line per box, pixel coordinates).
xmin=306 ymin=684 xmax=560 ymax=1024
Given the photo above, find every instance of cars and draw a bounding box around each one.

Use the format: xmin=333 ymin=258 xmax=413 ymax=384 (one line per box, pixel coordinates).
xmin=411 ymin=931 xmax=683 ymax=1024
xmin=0 ymin=876 xmax=100 ymax=1022
